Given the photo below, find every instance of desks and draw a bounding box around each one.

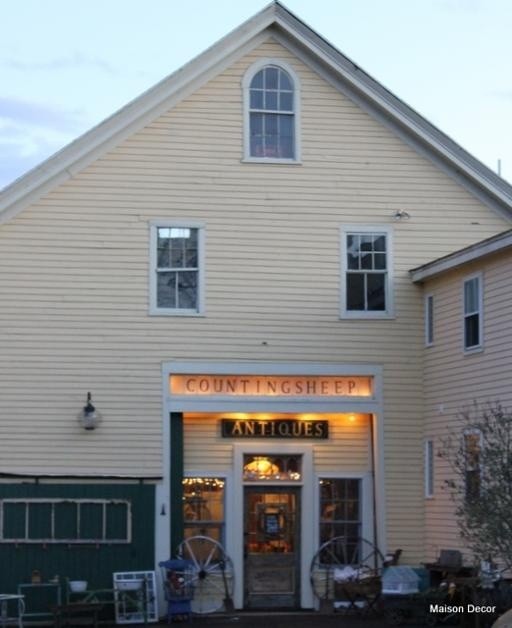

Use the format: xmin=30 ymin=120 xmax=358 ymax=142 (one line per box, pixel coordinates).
xmin=0 ymin=593 xmax=26 ymax=627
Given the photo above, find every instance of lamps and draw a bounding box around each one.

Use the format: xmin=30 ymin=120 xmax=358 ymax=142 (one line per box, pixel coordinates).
xmin=77 ymin=391 xmax=102 ymax=431
